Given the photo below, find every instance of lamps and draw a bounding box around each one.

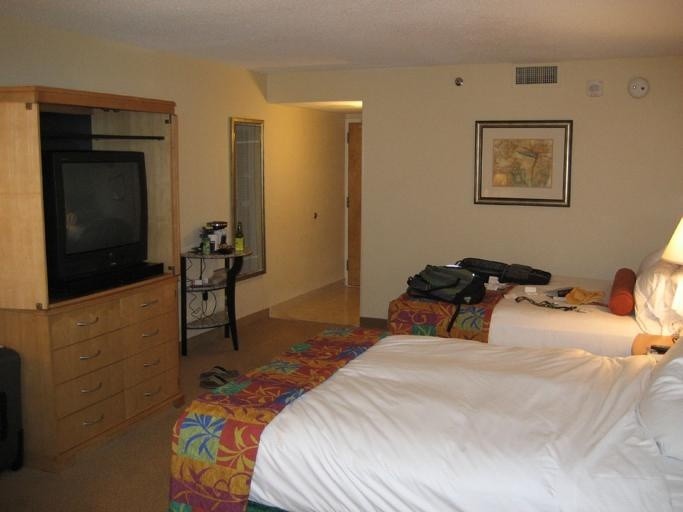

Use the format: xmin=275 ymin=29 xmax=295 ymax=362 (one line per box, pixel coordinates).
xmin=661 ymin=217 xmax=683 ymax=265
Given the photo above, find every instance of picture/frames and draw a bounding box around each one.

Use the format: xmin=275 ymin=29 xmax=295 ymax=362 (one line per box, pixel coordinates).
xmin=473 ymin=120 xmax=573 ymax=209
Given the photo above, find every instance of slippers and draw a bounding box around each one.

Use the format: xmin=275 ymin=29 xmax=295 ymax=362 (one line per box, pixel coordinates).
xmin=199 ymin=366 xmax=239 ymax=388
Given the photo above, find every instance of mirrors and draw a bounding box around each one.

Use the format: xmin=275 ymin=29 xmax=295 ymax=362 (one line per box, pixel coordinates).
xmin=230 ymin=117 xmax=267 ymax=282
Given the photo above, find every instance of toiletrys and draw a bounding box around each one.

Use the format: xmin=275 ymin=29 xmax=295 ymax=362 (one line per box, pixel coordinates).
xmin=202 ymin=221 xmax=244 ymax=255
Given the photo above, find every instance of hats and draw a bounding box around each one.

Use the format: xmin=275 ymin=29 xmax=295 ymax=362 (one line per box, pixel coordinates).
xmin=565 ymin=288 xmax=606 ymax=305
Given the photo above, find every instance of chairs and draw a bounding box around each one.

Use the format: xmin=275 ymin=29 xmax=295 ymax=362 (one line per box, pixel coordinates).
xmin=180 ymin=249 xmax=254 ymax=356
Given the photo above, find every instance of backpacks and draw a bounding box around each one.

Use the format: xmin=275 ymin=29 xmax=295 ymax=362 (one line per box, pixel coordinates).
xmin=406 ymin=264 xmax=486 ymax=305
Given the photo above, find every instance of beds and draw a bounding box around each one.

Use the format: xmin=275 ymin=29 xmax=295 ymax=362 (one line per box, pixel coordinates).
xmin=637 ymin=334 xmax=682 ymax=457
xmin=386 ymin=278 xmax=679 ymax=355
xmin=170 ymin=328 xmax=682 ymax=512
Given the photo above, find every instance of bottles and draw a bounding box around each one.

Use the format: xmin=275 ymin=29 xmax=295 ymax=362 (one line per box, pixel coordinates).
xmin=201 ymin=222 xmax=228 ymax=254
xmin=235 ymin=221 xmax=245 ymax=253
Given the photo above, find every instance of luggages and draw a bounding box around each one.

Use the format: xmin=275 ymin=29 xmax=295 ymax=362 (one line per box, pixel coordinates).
xmin=0 ymin=347 xmax=23 ymax=471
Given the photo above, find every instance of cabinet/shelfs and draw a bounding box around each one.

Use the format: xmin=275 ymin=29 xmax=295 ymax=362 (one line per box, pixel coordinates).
xmin=1 ymin=84 xmax=184 ymax=475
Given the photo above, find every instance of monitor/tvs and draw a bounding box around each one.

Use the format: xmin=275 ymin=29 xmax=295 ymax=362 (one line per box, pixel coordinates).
xmin=39 ymin=149 xmax=148 ymax=287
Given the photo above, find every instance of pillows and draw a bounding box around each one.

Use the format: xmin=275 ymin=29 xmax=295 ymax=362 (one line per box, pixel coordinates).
xmin=608 ymin=246 xmax=682 ymax=335
